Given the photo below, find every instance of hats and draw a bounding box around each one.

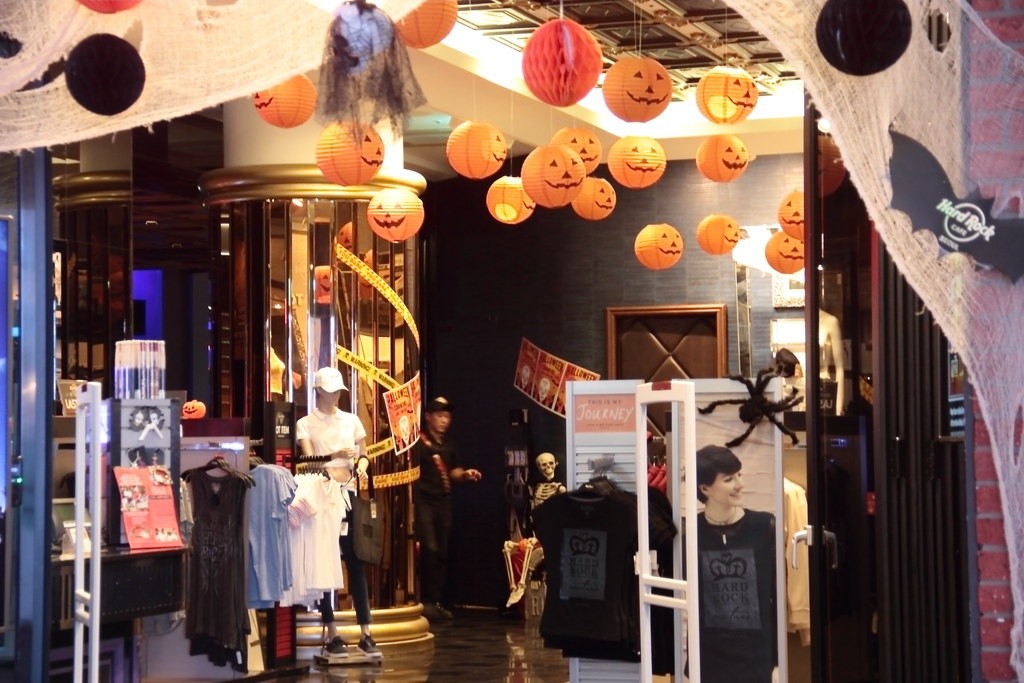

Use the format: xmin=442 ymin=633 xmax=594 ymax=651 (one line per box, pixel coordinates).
xmin=425 ymin=395 xmax=453 ymax=414
xmin=768 ymin=349 xmax=800 ymax=378
xmin=313 ymin=367 xmax=349 ymax=392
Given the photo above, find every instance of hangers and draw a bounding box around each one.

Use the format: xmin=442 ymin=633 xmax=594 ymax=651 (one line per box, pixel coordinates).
xmin=181 ymin=453 xmax=257 ymax=490
xmin=248 ymin=439 xmax=268 ymax=465
xmin=568 ymin=457 xmax=620 ymax=500
xmin=297 ymin=454 xmax=330 ymax=479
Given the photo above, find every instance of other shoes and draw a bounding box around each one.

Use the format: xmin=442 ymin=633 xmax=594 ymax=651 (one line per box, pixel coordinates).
xmin=422 ymin=603 xmax=451 ymax=620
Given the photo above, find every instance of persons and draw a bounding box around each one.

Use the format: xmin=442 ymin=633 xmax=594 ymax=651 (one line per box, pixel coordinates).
xmin=817 ymin=309 xmax=845 ymax=418
xmin=681 ymin=445 xmax=778 ymax=683
xmin=293 ymin=366 xmax=381 ymax=658
xmin=412 ymin=396 xmax=482 ymax=618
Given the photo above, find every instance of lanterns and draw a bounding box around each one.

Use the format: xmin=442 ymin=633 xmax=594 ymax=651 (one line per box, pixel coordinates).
xmin=184 ymin=400 xmax=207 ymax=418
xmin=65 ymin=0 xmax=806 ymax=275
xmin=815 ymin=0 xmax=912 ymax=76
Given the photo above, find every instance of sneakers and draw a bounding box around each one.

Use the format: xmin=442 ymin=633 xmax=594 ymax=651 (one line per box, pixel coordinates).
xmin=357 ymin=633 xmax=383 ymax=657
xmin=325 ymin=636 xmax=349 ymax=657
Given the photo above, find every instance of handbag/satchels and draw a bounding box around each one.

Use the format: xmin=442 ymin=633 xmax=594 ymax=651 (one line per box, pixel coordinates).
xmin=351 ymin=457 xmax=383 ymax=563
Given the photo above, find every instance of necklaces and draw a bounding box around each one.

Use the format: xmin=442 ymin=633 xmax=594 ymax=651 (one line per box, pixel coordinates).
xmin=704 ymin=506 xmax=738 ymax=544
